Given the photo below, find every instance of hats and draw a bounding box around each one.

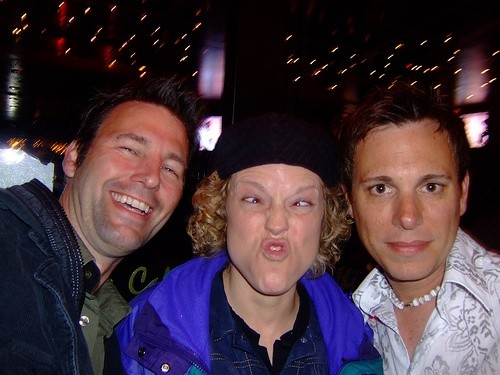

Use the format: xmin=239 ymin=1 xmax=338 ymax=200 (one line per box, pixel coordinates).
xmin=221 ymin=113 xmax=343 ymax=187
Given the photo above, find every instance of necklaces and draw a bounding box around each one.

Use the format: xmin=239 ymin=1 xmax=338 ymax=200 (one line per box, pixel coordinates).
xmin=388 ymin=284 xmax=442 ymax=310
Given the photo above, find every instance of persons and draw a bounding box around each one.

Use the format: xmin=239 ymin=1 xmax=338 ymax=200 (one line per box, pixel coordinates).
xmin=113 ymin=105 xmax=383 ymax=375
xmin=335 ymin=84 xmax=500 ymax=375
xmin=0 ymin=75 xmax=202 ymax=375
